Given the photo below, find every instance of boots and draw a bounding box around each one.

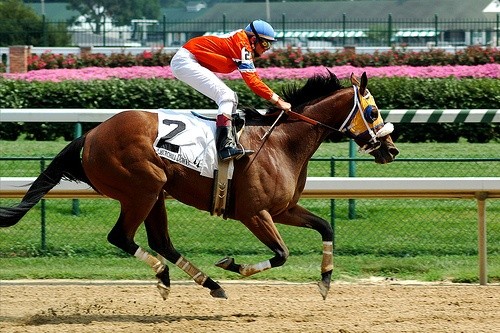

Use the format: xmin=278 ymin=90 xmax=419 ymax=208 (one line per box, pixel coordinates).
xmin=216 ymin=125 xmax=254 ymax=162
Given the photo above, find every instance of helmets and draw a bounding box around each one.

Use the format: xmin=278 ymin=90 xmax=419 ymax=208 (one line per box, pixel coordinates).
xmin=244 ymin=18 xmax=277 ymax=42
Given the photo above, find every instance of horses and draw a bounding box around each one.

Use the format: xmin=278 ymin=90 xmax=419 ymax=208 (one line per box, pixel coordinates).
xmin=0 ymin=68 xmax=401 ymax=302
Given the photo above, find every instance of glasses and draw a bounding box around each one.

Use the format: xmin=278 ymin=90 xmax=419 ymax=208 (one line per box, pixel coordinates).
xmin=257 ymin=37 xmax=271 ymax=51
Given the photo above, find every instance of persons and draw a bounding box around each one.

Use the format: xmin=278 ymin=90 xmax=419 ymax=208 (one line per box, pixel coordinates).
xmin=170 ymin=20 xmax=291 ymax=161
